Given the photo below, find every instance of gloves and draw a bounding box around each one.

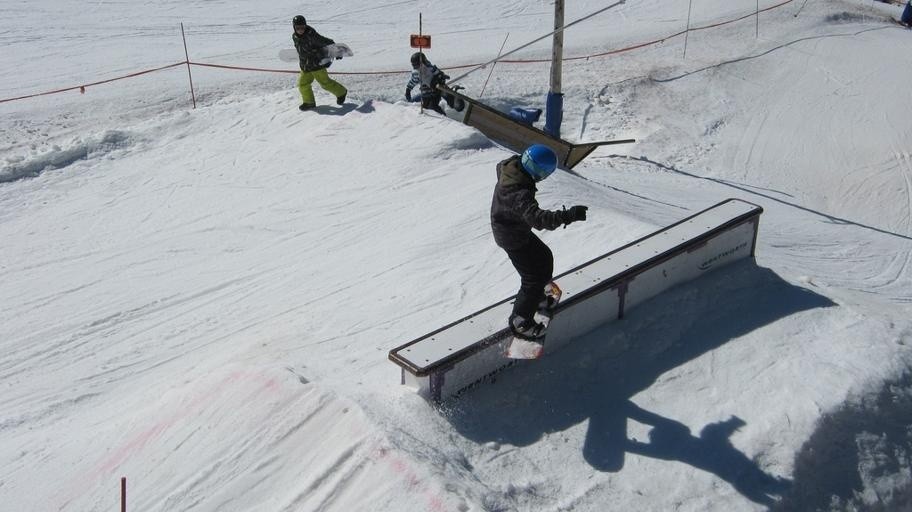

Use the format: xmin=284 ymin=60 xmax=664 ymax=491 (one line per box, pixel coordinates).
xmin=560 ymin=205 xmax=589 ymax=224
xmin=405 ymin=88 xmax=412 ymax=101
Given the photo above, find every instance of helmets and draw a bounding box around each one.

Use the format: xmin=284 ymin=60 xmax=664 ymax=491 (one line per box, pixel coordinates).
xmin=292 ymin=15 xmax=306 ymax=28
xmin=410 ymin=52 xmax=426 ymax=65
xmin=520 ymin=143 xmax=557 ymax=183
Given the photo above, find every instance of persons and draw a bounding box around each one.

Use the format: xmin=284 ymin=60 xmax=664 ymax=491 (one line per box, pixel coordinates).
xmin=488 ymin=143 xmax=589 ymax=344
xmin=404 ymin=49 xmax=450 ymax=119
xmin=291 ymin=14 xmax=349 ymax=112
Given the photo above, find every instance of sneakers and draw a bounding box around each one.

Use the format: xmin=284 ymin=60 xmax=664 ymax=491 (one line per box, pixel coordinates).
xmin=337 ymin=88 xmax=347 ymax=104
xmin=537 ymin=295 xmax=555 ymax=310
xmin=508 ymin=312 xmax=546 ymax=340
xmin=299 ymin=103 xmax=316 ymax=110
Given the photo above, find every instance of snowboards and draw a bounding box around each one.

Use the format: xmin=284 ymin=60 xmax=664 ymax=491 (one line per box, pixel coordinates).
xmin=420 ymin=65 xmax=464 ymax=112
xmin=279 ymin=41 xmax=353 ymax=61
xmin=506 ymin=282 xmax=561 ymax=360
